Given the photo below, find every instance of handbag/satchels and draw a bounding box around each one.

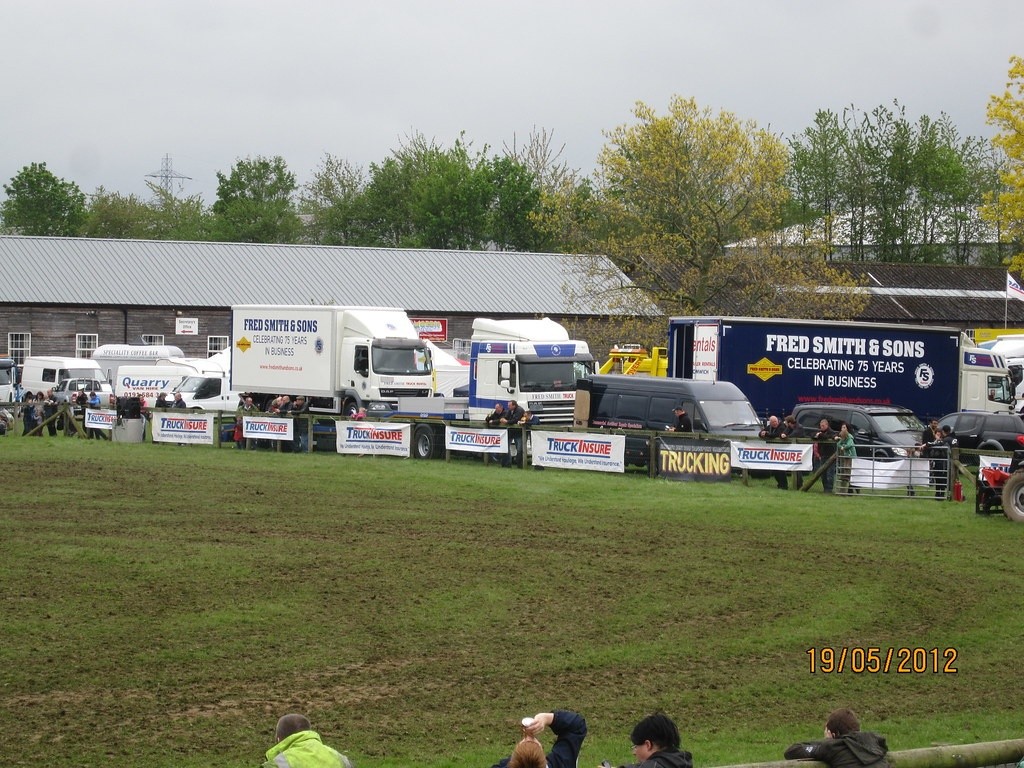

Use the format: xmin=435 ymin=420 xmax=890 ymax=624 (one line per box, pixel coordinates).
xmin=234 ymin=425 xmax=244 ymax=441
xmin=57 ymin=414 xmax=64 ymax=430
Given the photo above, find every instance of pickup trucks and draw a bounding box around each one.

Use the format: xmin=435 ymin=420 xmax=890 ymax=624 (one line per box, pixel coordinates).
xmin=51 ymin=377 xmax=115 ymax=417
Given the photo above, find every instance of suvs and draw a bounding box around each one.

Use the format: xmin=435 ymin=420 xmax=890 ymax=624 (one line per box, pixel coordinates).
xmin=936 ymin=409 xmax=1024 ymax=452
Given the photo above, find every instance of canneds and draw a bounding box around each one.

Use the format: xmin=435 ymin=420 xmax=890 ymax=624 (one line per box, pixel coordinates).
xmin=521 ymin=717 xmax=533 ymax=735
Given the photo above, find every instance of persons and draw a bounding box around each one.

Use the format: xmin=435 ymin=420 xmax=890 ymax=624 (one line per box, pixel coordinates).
xmin=351 ymin=407 xmax=366 ymax=458
xmin=784 ymin=708 xmax=890 ymax=768
xmin=21 ymin=388 xmax=108 ymax=440
xmin=115 ymin=394 xmax=149 ymax=441
xmin=171 ymin=393 xmax=186 ymax=446
xmin=597 ymin=714 xmax=693 ymax=768
xmin=485 ymin=400 xmax=545 ymax=470
xmin=260 ymin=714 xmax=354 ymax=768
xmin=233 ymin=395 xmax=311 ymax=452
xmin=837 ymin=424 xmax=857 ymax=496
xmin=491 ymin=710 xmax=587 ymax=768
xmin=664 ymin=406 xmax=692 ymax=432
xmin=811 ymin=419 xmax=837 ymax=492
xmin=914 ymin=419 xmax=957 ymax=501
xmin=759 ymin=415 xmax=789 ymax=490
xmin=780 ymin=415 xmax=808 ymax=490
xmin=152 ymin=392 xmax=168 ymax=445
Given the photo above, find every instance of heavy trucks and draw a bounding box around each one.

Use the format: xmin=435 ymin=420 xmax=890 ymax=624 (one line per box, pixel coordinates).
xmin=228 ymin=303 xmax=600 ymax=462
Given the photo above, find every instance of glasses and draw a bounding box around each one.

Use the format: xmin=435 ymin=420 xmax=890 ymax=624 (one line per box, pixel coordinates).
xmin=630 ymin=744 xmax=638 ymax=751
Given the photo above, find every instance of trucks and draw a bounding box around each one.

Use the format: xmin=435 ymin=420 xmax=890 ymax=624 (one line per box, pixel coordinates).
xmin=664 ymin=315 xmax=1024 ymax=427
xmin=0 ymin=342 xmax=231 ymax=417
xmin=230 ymin=305 xmax=438 ymax=418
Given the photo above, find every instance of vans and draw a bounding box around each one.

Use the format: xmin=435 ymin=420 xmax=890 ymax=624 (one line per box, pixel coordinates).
xmin=573 ymin=374 xmax=765 ymax=468
xmin=791 ymin=401 xmax=928 ymax=462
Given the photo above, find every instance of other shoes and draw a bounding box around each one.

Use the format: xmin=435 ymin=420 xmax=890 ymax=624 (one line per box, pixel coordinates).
xmin=935 ymin=493 xmax=944 ymax=501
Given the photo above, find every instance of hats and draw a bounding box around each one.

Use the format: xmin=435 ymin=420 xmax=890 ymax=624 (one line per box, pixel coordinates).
xmin=296 ymin=395 xmax=305 ymax=400
xmin=672 ymin=407 xmax=682 ymax=412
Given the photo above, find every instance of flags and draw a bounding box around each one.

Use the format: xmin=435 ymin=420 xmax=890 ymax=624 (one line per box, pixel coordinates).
xmin=1007 ymin=273 xmax=1024 ymax=301
